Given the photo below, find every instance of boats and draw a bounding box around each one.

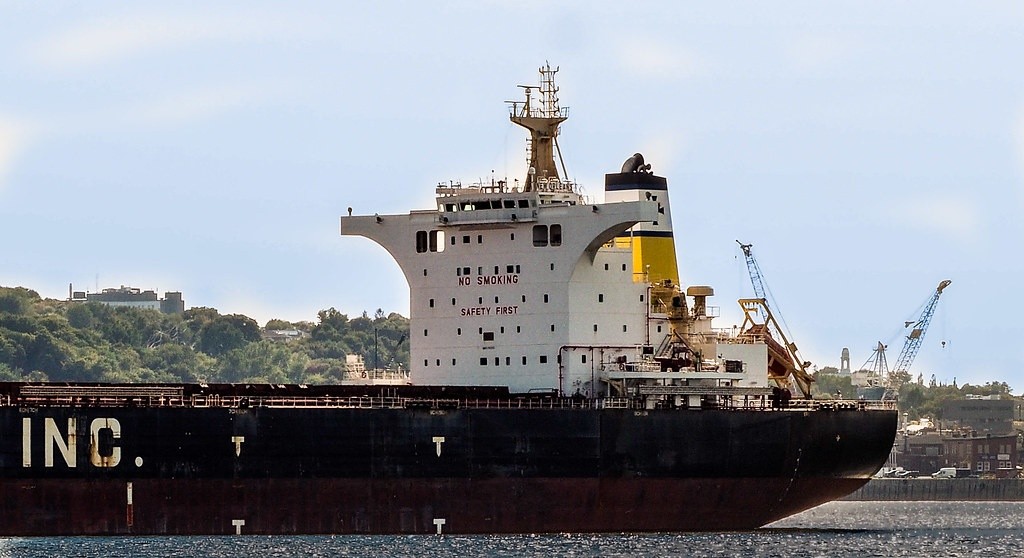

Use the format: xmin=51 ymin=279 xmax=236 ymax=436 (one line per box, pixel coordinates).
xmin=0 ymin=60 xmax=898 ymax=540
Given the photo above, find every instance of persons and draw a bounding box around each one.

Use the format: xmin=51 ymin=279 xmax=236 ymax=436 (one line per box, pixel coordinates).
xmin=772 ymin=385 xmax=781 ymax=411
xmin=781 ymin=385 xmax=791 ymax=411
xmin=692 ymin=351 xmax=700 ymax=371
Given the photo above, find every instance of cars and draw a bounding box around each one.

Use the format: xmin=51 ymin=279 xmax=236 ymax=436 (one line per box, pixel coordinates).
xmin=885 ymin=469 xmax=920 ymax=480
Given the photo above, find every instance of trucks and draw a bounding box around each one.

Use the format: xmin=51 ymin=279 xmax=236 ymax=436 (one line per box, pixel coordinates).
xmin=931 ymin=467 xmax=956 ymax=479
